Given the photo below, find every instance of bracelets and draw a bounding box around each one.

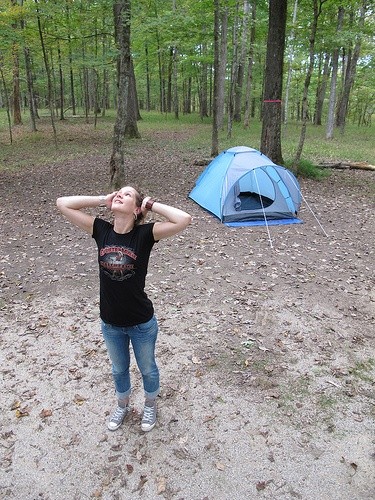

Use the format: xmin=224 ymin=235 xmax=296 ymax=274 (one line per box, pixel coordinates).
xmin=145 ymin=199 xmax=156 ymax=212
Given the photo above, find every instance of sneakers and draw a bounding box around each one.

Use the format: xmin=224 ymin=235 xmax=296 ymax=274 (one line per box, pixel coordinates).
xmin=141 ymin=401 xmax=158 ymax=432
xmin=107 ymin=403 xmax=130 ymax=431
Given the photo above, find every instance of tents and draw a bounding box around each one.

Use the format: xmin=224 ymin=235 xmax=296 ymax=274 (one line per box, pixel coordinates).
xmin=186 ymin=145 xmax=305 ymax=226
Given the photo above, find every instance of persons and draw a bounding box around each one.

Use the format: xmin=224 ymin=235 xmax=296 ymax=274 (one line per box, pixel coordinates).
xmin=56 ymin=185 xmax=192 ymax=431
